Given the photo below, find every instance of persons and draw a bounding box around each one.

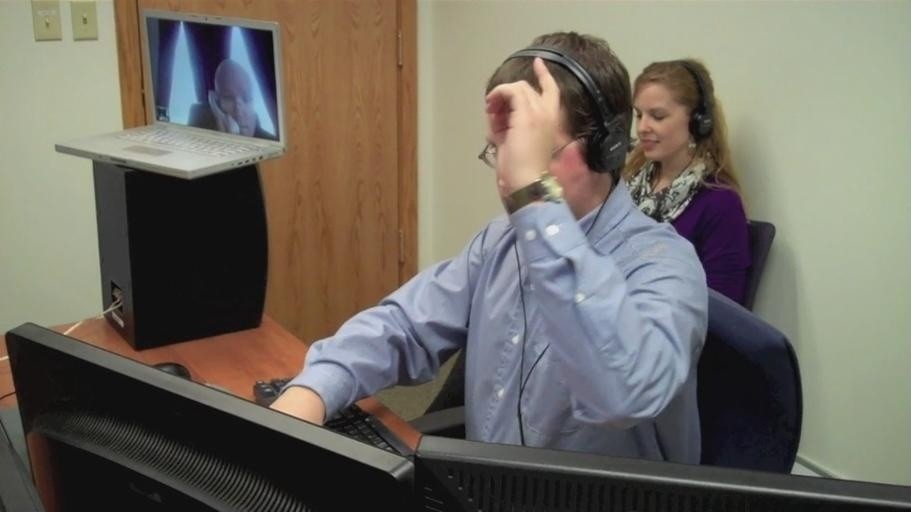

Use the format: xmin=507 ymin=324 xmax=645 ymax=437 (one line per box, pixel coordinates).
xmin=622 ymin=58 xmax=751 ymax=306
xmin=185 ymin=58 xmax=278 ymax=143
xmin=267 ymin=30 xmax=710 ymax=468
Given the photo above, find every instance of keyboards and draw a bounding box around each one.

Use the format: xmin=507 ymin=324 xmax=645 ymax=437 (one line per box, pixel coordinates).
xmin=253 ymin=378 xmax=414 ymax=458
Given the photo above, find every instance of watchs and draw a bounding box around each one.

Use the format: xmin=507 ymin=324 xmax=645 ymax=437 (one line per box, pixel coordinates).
xmin=500 ymin=168 xmax=565 ymax=213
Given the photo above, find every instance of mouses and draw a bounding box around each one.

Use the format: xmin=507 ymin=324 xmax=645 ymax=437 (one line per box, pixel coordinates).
xmin=153 ymin=363 xmax=191 ymax=380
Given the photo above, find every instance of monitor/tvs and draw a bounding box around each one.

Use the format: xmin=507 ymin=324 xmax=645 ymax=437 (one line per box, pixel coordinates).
xmin=414 ymin=436 xmax=911 ymax=512
xmin=5 ymin=323 xmax=414 ymax=512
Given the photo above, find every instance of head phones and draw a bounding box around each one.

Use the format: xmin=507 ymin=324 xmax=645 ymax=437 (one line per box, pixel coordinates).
xmin=679 ymin=60 xmax=714 ymax=140
xmin=503 ymin=47 xmax=627 ymax=172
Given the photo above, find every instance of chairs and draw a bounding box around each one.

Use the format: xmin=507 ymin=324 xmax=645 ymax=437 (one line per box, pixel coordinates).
xmin=743 ymin=219 xmax=777 ymax=310
xmin=408 ymin=288 xmax=802 ymax=474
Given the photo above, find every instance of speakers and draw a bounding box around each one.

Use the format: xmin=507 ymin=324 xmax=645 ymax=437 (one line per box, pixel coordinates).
xmin=93 ymin=159 xmax=268 ymax=351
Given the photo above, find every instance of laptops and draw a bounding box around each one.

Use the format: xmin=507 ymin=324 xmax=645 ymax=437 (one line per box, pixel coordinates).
xmin=55 ymin=9 xmax=286 ymax=180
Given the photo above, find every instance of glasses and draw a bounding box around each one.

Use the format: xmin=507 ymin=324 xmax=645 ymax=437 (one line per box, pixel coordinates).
xmin=479 ymin=141 xmax=573 ymax=170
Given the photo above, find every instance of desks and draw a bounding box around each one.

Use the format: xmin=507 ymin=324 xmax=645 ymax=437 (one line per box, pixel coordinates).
xmin=0 ymin=315 xmax=423 ymax=452
